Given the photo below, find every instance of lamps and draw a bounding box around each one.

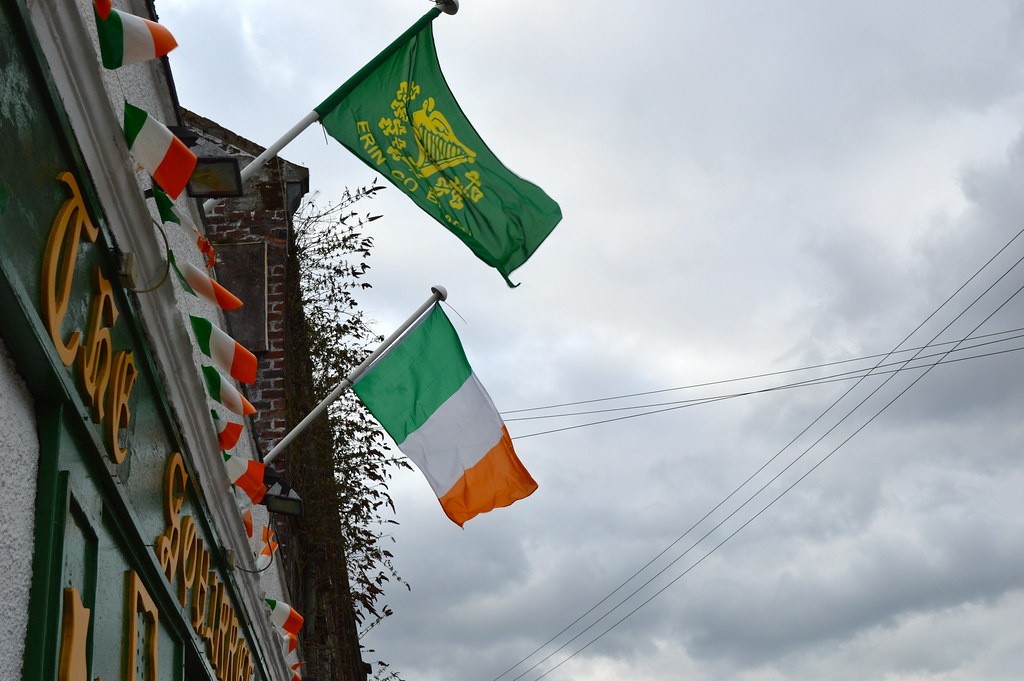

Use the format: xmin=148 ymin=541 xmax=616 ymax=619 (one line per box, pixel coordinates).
xmin=257 ymin=480 xmax=305 ymax=519
xmin=144 ymin=156 xmax=244 ymax=201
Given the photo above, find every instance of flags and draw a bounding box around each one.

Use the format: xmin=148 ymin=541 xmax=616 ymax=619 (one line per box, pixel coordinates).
xmin=316 ymin=22 xmax=562 ymax=277
xmin=351 ymin=302 xmax=538 ymax=528
xmin=94 ymin=0 xmax=307 ymax=681
